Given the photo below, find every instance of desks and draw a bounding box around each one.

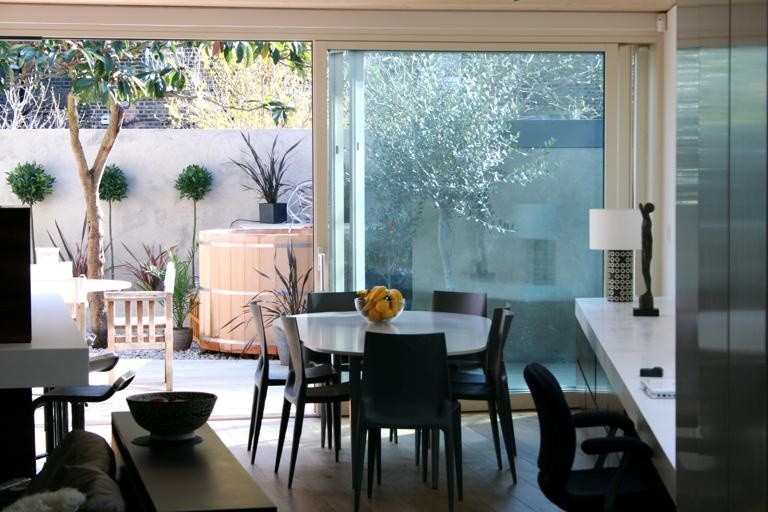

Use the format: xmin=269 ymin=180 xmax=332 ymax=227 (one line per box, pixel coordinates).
xmin=52 ymin=278 xmax=132 ymax=342
xmin=574 ymin=297 xmax=677 ymax=504
xmin=0 ymin=344 xmax=89 ymax=456
xmin=111 ymin=412 xmax=278 ymax=512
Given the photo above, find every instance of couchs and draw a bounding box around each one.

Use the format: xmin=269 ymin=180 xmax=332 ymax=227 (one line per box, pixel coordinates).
xmin=1 ymin=429 xmax=125 ymax=512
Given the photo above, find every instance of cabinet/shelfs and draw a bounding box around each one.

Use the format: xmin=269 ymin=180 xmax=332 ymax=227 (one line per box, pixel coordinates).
xmin=198 ymin=223 xmax=313 ymax=356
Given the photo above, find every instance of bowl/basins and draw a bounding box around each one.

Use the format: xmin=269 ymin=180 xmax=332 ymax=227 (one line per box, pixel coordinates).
xmin=354 ymin=298 xmax=406 ymax=324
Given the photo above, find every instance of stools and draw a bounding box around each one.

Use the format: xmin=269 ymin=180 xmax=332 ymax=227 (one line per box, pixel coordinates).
xmin=27 ymin=371 xmax=135 ymax=478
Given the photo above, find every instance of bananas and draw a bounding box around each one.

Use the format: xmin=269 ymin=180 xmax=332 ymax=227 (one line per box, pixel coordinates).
xmin=388 ymin=288 xmax=403 ymax=317
xmin=360 ymin=285 xmax=388 ymax=317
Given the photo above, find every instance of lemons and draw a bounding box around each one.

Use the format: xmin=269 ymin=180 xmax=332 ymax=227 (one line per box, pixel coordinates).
xmin=368 ymin=307 xmax=380 ymax=321
xmin=381 ymin=308 xmax=393 ymax=320
xmin=376 ymin=299 xmax=389 ymax=313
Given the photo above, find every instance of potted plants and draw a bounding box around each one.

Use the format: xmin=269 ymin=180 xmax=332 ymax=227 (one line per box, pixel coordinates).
xmin=141 ymin=244 xmax=203 ymax=353
xmin=220 ymin=233 xmax=313 ymax=366
xmin=222 ymin=130 xmax=304 ymax=223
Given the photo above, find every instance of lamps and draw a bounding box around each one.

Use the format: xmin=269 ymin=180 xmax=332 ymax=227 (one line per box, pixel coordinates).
xmin=588 ymin=209 xmax=643 ymax=302
xmin=507 ymin=203 xmax=559 ymax=289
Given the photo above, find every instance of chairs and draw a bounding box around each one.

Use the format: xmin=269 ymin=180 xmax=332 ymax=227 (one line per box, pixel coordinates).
xmin=523 ymin=362 xmax=676 ymax=511
xmin=104 ymin=260 xmax=176 ymax=392
